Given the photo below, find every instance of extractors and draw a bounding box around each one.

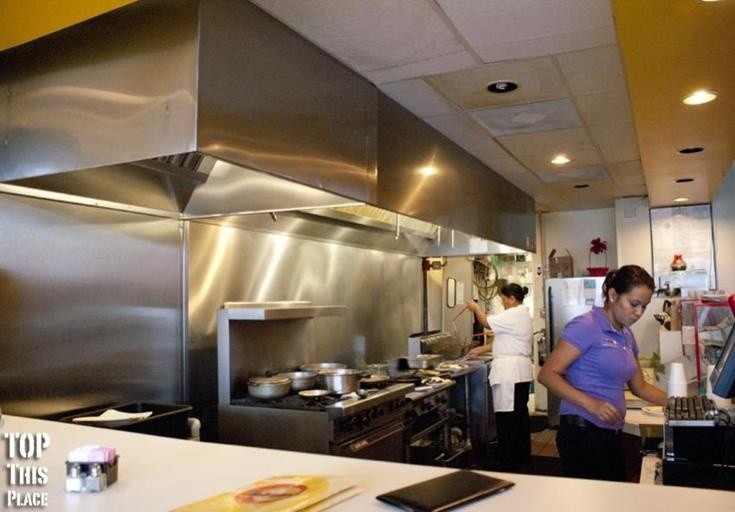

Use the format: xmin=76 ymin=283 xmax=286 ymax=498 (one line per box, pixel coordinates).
xmin=187 ymin=86 xmax=538 ymax=258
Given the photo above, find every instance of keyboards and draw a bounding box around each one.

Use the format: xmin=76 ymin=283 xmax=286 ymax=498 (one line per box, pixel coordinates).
xmin=664 ymin=395 xmax=717 ymax=427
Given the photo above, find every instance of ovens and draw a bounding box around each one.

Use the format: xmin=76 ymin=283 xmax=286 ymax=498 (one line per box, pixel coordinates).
xmin=402 ymin=388 xmax=452 ymax=468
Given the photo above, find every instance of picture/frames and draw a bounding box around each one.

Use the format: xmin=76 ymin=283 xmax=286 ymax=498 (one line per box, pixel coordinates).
xmin=649 ymin=202 xmax=718 ymax=294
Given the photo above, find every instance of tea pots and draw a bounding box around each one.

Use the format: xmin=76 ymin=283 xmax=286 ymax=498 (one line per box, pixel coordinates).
xmin=671 ymin=254 xmax=687 ymax=271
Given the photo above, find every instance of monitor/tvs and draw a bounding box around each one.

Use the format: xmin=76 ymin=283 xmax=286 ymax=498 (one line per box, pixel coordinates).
xmin=710 ymin=323 xmax=734 ymax=402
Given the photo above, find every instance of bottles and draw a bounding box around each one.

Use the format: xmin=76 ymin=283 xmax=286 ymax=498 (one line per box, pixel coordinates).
xmin=64 ymin=462 xmax=108 ymax=494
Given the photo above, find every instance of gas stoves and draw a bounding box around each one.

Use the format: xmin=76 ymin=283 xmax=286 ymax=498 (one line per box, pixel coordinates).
xmin=230 ymin=388 xmax=380 ymax=411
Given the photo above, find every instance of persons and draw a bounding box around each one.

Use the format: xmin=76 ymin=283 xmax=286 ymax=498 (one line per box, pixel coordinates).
xmin=463 ymin=283 xmax=535 ymax=472
xmin=537 ymin=264 xmax=667 ymax=483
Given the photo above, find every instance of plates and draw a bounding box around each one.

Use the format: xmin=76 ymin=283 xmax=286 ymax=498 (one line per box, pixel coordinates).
xmin=641 ymin=405 xmax=666 ymax=416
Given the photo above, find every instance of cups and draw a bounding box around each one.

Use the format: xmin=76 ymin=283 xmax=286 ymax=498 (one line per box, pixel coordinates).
xmin=666 ymin=363 xmax=688 ymax=398
xmin=682 ymin=325 xmax=696 ymax=357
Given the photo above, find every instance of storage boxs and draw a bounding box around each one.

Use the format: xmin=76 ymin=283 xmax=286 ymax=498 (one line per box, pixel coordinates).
xmin=549 ymin=249 xmax=573 ymax=278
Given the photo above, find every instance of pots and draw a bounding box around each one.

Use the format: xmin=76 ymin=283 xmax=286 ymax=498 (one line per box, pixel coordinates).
xmin=246 ymin=362 xmax=363 ymax=400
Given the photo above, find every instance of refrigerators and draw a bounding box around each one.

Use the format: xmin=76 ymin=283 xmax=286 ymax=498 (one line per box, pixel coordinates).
xmin=544 ymin=276 xmax=607 ymax=427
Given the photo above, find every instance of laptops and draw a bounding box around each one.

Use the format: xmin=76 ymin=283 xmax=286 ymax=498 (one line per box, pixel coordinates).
xmin=375 ymin=466 xmax=516 ymax=511
